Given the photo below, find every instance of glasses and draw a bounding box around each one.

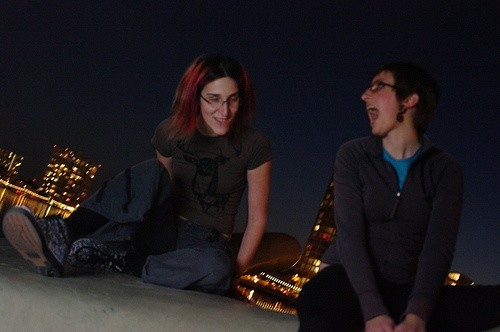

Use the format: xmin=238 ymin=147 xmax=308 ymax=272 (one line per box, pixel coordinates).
xmin=201 ymin=93 xmax=239 ymax=104
xmin=370 ymin=80 xmax=395 ymax=93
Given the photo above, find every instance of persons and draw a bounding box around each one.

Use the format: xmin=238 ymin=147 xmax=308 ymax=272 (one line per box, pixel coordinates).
xmin=295 ymin=61 xmax=500 ymax=332
xmin=3 ymin=52 xmax=272 ymax=298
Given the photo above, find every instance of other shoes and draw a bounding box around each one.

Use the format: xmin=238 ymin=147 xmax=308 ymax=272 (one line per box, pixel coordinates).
xmin=2 ymin=207 xmax=71 ymax=275
xmin=69 ymin=237 xmax=124 ymax=273
